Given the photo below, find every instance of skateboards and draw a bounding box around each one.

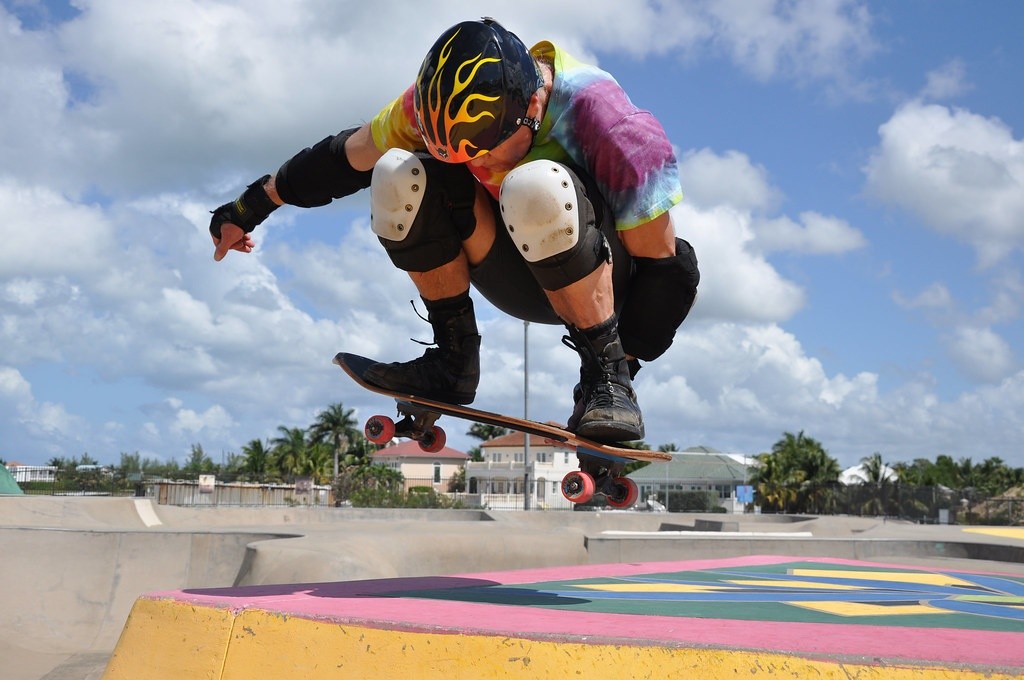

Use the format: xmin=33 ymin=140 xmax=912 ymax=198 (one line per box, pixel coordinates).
xmin=333 ymin=349 xmax=672 ymax=510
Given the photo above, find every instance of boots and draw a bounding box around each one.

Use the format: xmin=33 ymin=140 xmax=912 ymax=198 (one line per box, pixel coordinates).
xmin=362 ymin=297 xmax=482 ymax=405
xmin=561 ymin=323 xmax=645 ymax=441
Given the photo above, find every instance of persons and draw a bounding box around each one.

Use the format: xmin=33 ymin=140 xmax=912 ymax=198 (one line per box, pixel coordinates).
xmin=208 ymin=15 xmax=700 ymax=440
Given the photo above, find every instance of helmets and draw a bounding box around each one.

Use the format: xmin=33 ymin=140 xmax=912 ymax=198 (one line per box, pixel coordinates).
xmin=413 ymin=17 xmax=545 ymax=163
